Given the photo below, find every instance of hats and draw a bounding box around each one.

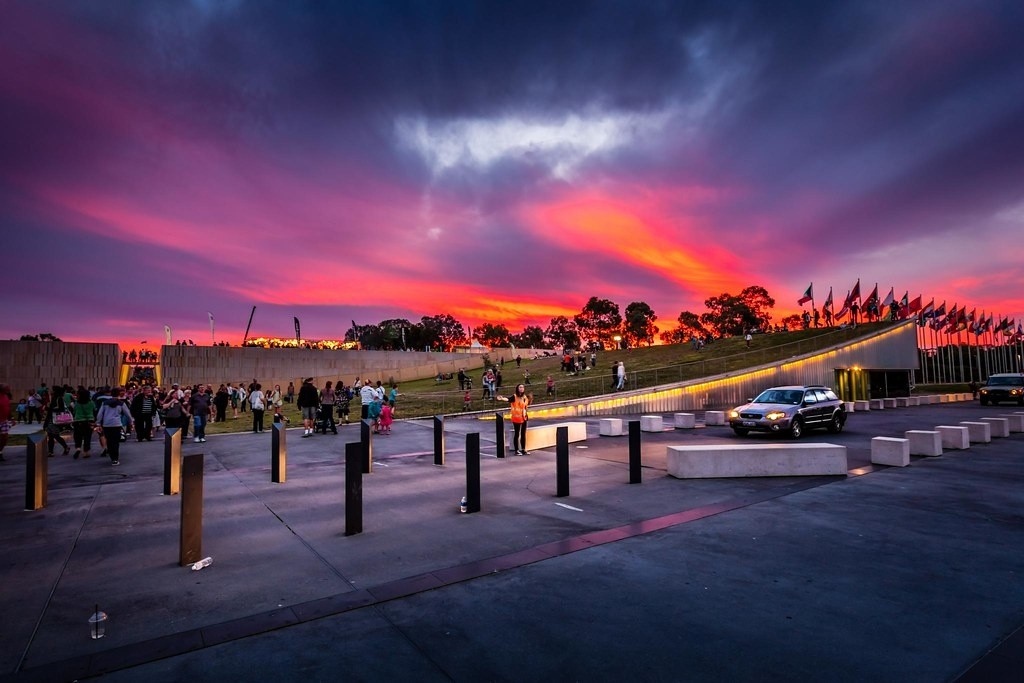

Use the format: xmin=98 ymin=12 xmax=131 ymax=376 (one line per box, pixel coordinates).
xmin=365 ymin=379 xmax=372 ymax=385
xmin=173 ymin=383 xmax=179 ymax=385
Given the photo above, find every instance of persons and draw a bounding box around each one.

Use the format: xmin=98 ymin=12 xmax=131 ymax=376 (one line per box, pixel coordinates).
xmin=434 ymin=340 xmax=628 ymax=412
xmin=497 ymin=384 xmax=533 ymax=456
xmin=1 ymin=339 xmax=405 ymax=466
xmin=690 ymin=300 xmax=899 ymax=353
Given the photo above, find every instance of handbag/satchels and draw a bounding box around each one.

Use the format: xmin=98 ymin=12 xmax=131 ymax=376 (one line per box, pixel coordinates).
xmin=255 ymin=391 xmax=265 ymax=411
xmin=88 ymin=421 xmax=96 ymax=429
xmin=52 ymin=397 xmax=73 ymax=424
xmin=383 ymin=395 xmax=389 ymax=401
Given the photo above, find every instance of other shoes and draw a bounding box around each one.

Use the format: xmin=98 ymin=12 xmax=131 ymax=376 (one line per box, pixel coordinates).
xmin=194 ymin=437 xmax=206 ymax=443
xmin=386 ymin=432 xmax=390 ymax=435
xmin=301 ymin=433 xmax=309 ymax=437
xmin=514 ymin=450 xmax=523 ymax=456
xmin=337 ymin=424 xmax=342 ymax=426
xmin=522 ymin=451 xmax=531 ymax=455
xmin=379 ymin=432 xmax=384 ymax=435
xmin=47 ymin=445 xmax=120 ymax=466
xmin=308 ymin=432 xmax=313 ymax=436
xmin=345 ymin=419 xmax=349 ymax=424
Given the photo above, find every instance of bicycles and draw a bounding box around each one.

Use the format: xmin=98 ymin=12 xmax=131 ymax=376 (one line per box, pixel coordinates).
xmin=465 ymin=376 xmax=472 ymax=391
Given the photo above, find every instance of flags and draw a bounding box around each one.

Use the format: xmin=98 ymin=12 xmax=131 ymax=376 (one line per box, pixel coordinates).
xmin=796 ymin=281 xmax=1024 ymax=343
xmin=208 ymin=312 xmax=214 ymax=340
xmin=165 ymin=325 xmax=171 ymax=345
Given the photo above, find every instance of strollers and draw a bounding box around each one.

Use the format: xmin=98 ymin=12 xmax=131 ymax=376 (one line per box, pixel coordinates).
xmin=314 ymin=405 xmax=333 ymax=432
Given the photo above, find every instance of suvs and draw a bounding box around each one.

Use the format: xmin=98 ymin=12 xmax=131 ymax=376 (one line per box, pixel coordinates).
xmin=980 ymin=373 xmax=1024 ymax=405
xmin=729 ymin=386 xmax=847 ymax=436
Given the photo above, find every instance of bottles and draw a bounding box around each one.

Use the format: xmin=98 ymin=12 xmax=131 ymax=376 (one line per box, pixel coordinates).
xmin=192 ymin=557 xmax=212 ymax=570
xmin=461 ymin=496 xmax=467 ymax=512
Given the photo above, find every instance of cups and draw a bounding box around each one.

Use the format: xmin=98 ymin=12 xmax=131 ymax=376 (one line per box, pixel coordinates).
xmin=89 ymin=616 xmax=107 ymax=639
